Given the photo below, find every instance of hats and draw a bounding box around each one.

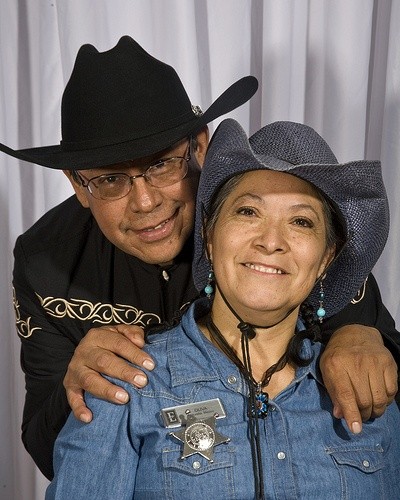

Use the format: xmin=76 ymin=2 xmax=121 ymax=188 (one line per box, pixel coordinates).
xmin=0 ymin=31 xmax=260 ymax=170
xmin=189 ymin=116 xmax=390 ymax=324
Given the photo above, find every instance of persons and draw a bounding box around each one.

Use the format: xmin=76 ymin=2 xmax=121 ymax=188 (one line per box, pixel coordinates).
xmin=45 ymin=114 xmax=399 ymax=500
xmin=10 ymin=31 xmax=399 ymax=484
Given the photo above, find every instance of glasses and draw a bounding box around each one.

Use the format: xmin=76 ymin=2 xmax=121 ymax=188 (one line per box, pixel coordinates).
xmin=70 ymin=137 xmax=195 ymax=200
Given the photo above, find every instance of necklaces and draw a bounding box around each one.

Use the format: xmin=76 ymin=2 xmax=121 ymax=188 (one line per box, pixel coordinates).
xmin=203 ymin=311 xmax=303 ymax=420
